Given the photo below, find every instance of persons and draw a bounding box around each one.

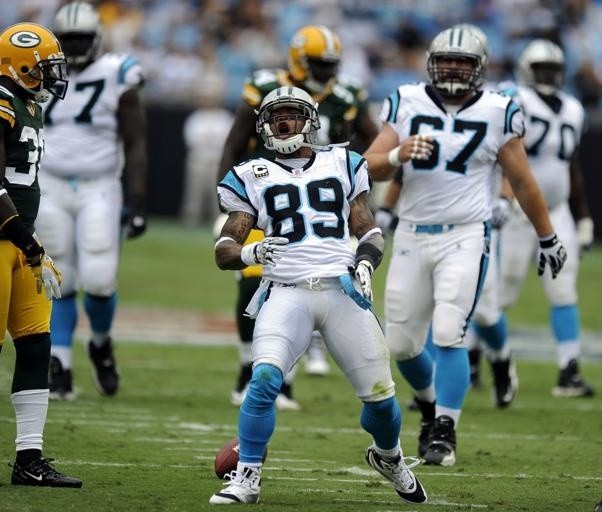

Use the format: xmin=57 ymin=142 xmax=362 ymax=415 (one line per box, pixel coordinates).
xmin=375 ymin=20 xmax=522 ymax=409
xmin=1 ymin=20 xmax=84 ymax=488
xmin=1 ymin=1 xmax=601 ymax=242
xmin=31 ymin=1 xmax=152 ymax=401
xmin=214 ymin=24 xmax=383 ymax=416
xmin=467 ymin=38 xmax=597 ymax=399
xmin=359 ymin=25 xmax=571 ymax=469
xmin=202 ymin=86 xmax=428 ymax=508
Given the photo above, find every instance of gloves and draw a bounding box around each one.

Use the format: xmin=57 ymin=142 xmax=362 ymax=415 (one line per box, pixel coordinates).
xmin=24 ymin=241 xmax=64 ymax=301
xmin=121 ymin=192 xmax=150 ymax=239
xmin=346 ymin=252 xmax=377 ymax=302
xmin=491 ymin=195 xmax=512 ymax=228
xmin=373 ymin=207 xmax=393 ymax=236
xmin=536 ymin=231 xmax=568 ymax=280
xmin=576 ymin=217 xmax=597 ymax=252
xmin=240 ymin=222 xmax=289 ymax=267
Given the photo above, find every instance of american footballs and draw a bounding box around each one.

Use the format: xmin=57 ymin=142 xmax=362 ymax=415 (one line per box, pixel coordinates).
xmin=215 ymin=438 xmax=268 ymax=480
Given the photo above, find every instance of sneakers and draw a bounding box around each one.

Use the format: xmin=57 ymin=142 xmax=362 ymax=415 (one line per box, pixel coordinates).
xmin=49 ymin=354 xmax=75 ymax=401
xmin=467 ymin=349 xmax=482 ymax=392
xmin=208 ymin=460 xmax=264 ymax=505
xmin=550 ymin=359 xmax=594 ymax=398
xmin=275 ymin=379 xmax=302 ymax=413
xmin=486 ymin=349 xmax=519 ymax=409
xmin=229 ymin=361 xmax=253 ymax=408
xmin=84 ymin=336 xmax=120 ymax=397
xmin=405 ymin=391 xmax=457 ymax=468
xmin=364 ymin=443 xmax=428 ymax=504
xmin=10 ymin=454 xmax=83 ymax=488
xmin=302 ymin=327 xmax=332 ymax=376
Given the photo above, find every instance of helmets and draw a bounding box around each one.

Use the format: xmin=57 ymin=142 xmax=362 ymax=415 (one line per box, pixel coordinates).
xmin=0 ymin=21 xmax=70 ymax=104
xmin=425 ymin=21 xmax=491 ymax=98
xmin=288 ymin=24 xmax=343 ymax=94
xmin=53 ymin=2 xmax=102 ymax=67
xmin=516 ymin=37 xmax=566 ymax=98
